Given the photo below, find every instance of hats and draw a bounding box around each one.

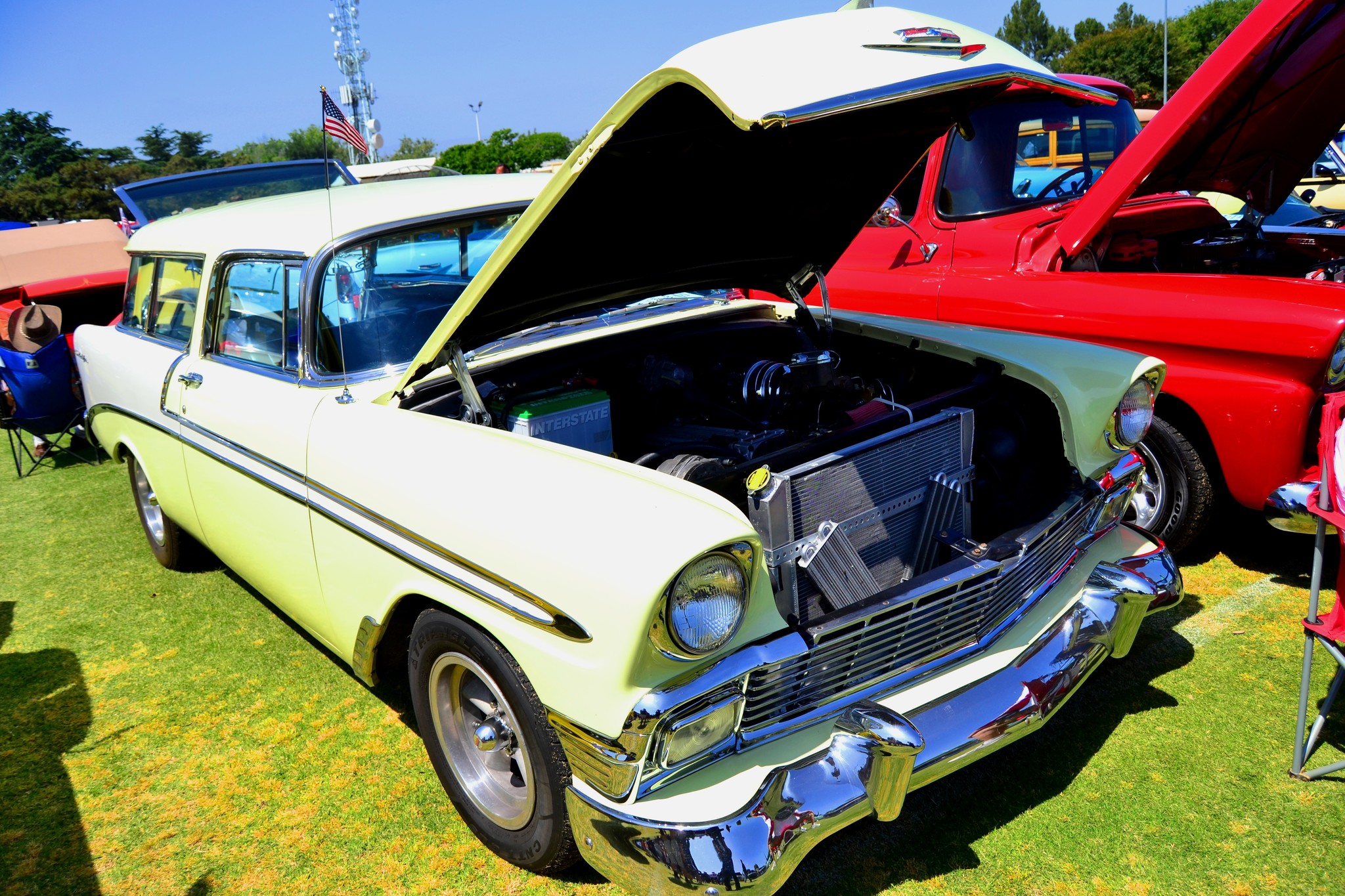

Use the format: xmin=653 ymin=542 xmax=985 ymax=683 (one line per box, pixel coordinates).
xmin=7 ymin=304 xmax=62 ymax=354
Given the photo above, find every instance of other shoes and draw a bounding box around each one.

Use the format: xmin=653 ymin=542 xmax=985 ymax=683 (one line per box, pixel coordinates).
xmin=33 ymin=441 xmax=50 ymax=458
xmin=70 ymin=434 xmax=98 ymax=449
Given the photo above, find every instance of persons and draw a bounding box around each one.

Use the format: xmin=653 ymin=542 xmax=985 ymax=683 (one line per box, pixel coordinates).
xmin=7 ymin=303 xmax=99 ymax=457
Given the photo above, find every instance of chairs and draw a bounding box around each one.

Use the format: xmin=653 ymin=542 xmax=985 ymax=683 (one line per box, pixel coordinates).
xmin=0 ymin=332 xmax=103 ymax=477
xmin=223 ymin=277 xmax=465 ymax=369
xmin=1289 ymin=391 xmax=1345 ymax=782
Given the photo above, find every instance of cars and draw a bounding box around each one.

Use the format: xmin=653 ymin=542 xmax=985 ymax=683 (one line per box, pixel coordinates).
xmin=724 ymin=1 xmax=1344 ymax=569
xmin=73 ymin=0 xmax=1185 ymax=895
xmin=0 ymin=157 xmax=515 ymax=417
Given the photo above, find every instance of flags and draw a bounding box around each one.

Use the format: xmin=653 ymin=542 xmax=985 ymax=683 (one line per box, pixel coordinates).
xmin=322 ymin=91 xmax=368 ymax=156
xmin=119 ymin=208 xmax=132 ymax=236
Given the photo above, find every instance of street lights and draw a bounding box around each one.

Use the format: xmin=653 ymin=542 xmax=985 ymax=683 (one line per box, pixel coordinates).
xmin=467 ymin=101 xmax=482 ymax=142
